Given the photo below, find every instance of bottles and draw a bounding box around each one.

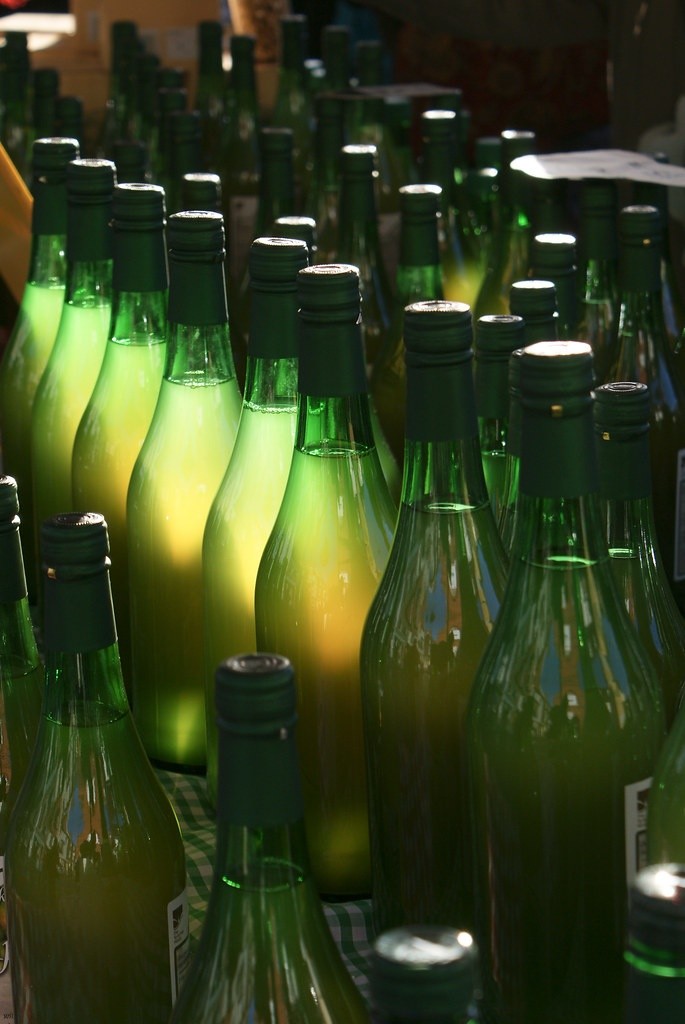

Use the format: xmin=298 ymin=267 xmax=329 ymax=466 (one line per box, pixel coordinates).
xmin=0 ymin=12 xmax=684 ymax=1024
